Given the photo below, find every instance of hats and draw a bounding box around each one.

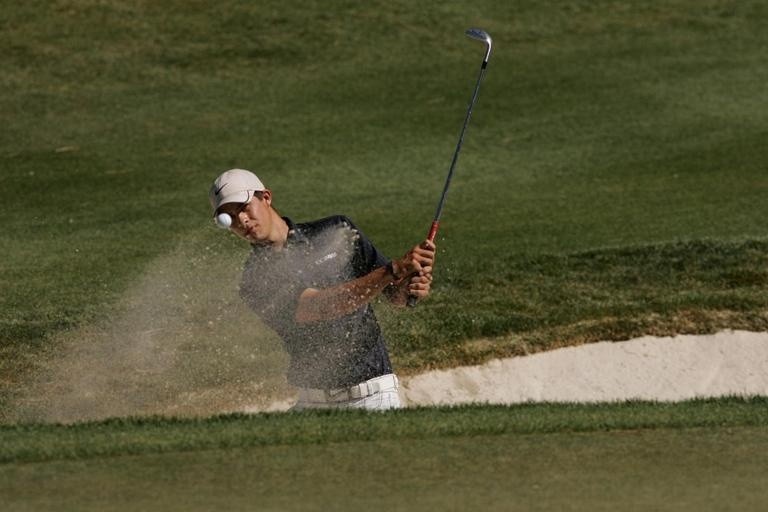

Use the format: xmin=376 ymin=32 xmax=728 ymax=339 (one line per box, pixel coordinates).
xmin=209 ymin=169 xmax=266 ymax=217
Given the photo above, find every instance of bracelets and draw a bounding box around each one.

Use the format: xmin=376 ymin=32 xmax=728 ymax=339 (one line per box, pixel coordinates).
xmin=386 ymin=260 xmax=400 ymax=281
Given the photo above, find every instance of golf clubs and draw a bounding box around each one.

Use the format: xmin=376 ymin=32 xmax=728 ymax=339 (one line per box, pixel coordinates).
xmin=407 ymin=27 xmax=491 ymax=310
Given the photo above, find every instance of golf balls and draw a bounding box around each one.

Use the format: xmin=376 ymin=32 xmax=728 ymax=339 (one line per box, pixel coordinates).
xmin=216 ymin=214 xmax=231 ymax=230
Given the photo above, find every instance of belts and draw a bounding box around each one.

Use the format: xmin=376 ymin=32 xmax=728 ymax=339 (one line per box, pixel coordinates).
xmin=297 ymin=373 xmax=399 ymax=403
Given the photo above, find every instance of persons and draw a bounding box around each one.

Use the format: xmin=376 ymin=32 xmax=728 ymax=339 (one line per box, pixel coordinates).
xmin=209 ymin=168 xmax=436 ymax=413
